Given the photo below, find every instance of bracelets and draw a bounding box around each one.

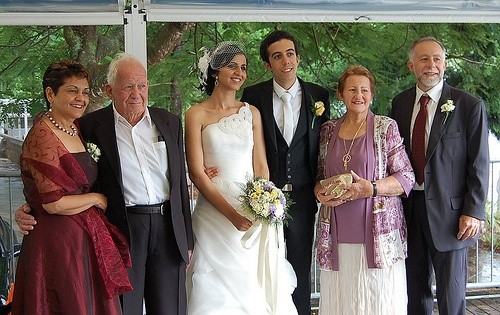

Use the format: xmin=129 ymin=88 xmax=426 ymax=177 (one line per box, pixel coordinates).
xmin=371 ymin=180 xmax=378 ymax=199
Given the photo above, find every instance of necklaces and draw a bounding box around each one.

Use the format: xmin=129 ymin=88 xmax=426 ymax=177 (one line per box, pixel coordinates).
xmin=342 ymin=115 xmax=366 ymax=170
xmin=44 ymin=110 xmax=77 ymax=137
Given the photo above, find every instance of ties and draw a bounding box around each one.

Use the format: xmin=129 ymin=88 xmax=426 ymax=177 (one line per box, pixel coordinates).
xmin=281 ymin=92 xmax=293 ymax=147
xmin=411 ymin=95 xmax=431 ymax=187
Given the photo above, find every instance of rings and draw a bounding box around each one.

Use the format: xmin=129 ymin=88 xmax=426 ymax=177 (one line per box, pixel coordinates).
xmin=350 ymin=198 xmax=353 ymax=201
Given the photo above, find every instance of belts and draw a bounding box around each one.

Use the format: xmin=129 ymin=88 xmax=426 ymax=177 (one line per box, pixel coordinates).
xmin=125 ymin=201 xmax=171 ymax=216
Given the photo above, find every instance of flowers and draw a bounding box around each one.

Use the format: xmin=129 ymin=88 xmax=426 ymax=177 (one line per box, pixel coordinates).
xmin=307 ymin=93 xmax=325 ymax=129
xmin=232 ymin=170 xmax=296 ymax=231
xmin=441 ymin=99 xmax=456 ymax=125
xmin=186 ymin=46 xmax=212 ymax=86
xmin=86 ymin=141 xmax=101 ymax=163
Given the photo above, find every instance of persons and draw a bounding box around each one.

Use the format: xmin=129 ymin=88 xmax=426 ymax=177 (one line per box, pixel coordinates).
xmin=11 ymin=54 xmax=133 ymax=315
xmin=390 ymin=37 xmax=489 ymax=315
xmin=15 ymin=53 xmax=195 ymax=315
xmin=241 ymin=31 xmax=332 ymax=315
xmin=315 ymin=64 xmax=417 ymax=315
xmin=183 ymin=41 xmax=299 ymax=315
